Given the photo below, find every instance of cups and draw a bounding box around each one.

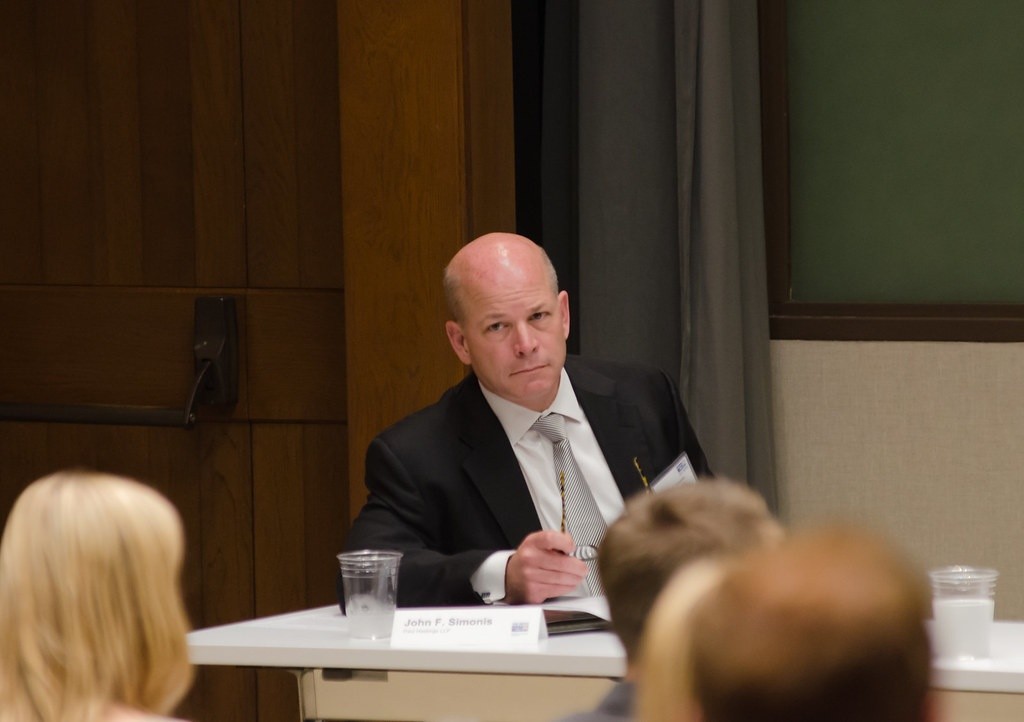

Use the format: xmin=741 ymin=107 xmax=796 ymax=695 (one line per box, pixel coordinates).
xmin=336 ymin=550 xmax=403 ymax=639
xmin=930 ymin=566 xmax=999 ymax=659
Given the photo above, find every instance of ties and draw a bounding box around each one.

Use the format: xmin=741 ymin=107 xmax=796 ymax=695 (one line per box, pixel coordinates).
xmin=528 ymin=414 xmax=611 ymax=596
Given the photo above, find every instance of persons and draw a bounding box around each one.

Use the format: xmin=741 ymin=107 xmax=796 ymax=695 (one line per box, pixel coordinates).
xmin=337 ymin=233 xmax=725 ymax=606
xmin=633 ymin=525 xmax=944 ymax=722
xmin=569 ymin=476 xmax=787 ymax=722
xmin=1 ymin=467 xmax=193 ymax=722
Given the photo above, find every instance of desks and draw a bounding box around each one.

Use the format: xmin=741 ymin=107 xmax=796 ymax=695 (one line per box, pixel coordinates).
xmin=185 ymin=605 xmax=1024 ymax=722
